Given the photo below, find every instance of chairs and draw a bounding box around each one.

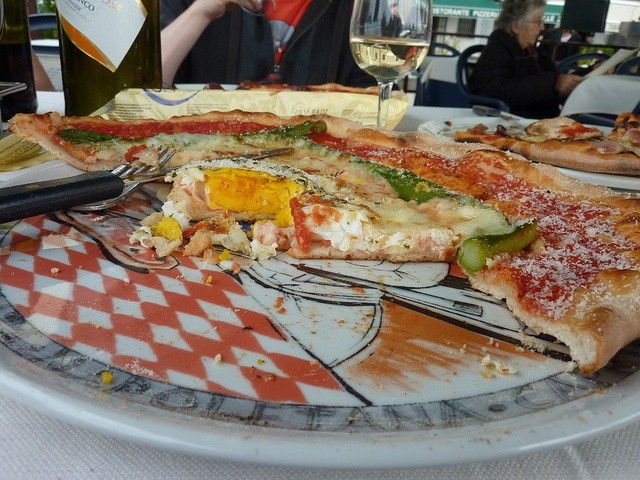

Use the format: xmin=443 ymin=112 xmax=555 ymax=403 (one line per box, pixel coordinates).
xmin=428 ymin=43 xmax=462 ymax=105
xmin=554 ymin=53 xmax=611 ymax=76
xmin=455 ymin=44 xmax=510 ymax=114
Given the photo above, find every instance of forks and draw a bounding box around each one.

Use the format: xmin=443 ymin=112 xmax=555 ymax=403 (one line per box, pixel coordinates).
xmin=69 ymin=146 xmax=180 ymax=212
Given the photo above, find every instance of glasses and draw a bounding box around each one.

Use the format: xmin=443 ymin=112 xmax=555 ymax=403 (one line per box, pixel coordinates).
xmin=524 ymin=17 xmax=547 ymax=27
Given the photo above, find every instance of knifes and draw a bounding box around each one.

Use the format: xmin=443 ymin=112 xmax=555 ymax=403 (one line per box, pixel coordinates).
xmin=1 ymin=144 xmax=294 ymax=225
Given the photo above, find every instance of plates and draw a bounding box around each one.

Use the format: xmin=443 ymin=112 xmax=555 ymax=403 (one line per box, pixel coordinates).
xmin=418 ymin=116 xmax=639 ymax=189
xmin=1 ymin=159 xmax=639 ymax=472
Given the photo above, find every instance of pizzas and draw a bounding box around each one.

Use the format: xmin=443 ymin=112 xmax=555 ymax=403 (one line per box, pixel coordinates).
xmin=234 ymin=82 xmax=379 ymax=95
xmin=9 ymin=109 xmax=640 ymax=376
xmin=453 ymin=106 xmax=640 ymax=180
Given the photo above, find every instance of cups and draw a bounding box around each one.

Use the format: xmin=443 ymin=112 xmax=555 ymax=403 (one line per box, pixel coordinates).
xmin=349 ymin=0 xmax=434 ymax=128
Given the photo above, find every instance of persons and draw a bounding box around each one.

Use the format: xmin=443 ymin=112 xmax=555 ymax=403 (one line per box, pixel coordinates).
xmin=466 ymin=1 xmax=584 ymax=119
xmin=159 ymin=0 xmax=400 ymax=91
xmin=381 ymin=4 xmax=402 ymax=38
